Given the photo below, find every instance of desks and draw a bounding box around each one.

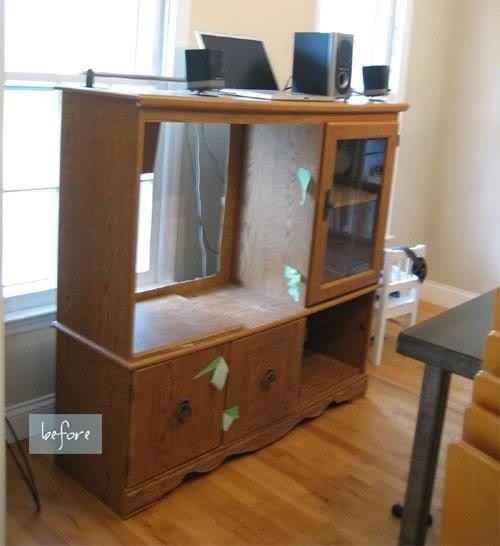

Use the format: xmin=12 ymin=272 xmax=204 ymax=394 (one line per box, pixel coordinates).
xmin=394 ymin=280 xmax=500 ymax=544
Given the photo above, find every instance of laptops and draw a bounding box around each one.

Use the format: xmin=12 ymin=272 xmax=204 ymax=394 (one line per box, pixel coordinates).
xmin=194 ymin=29 xmax=336 ymax=101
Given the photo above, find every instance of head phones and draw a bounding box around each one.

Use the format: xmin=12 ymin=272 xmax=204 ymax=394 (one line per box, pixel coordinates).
xmin=394 ymin=244 xmax=428 ymax=283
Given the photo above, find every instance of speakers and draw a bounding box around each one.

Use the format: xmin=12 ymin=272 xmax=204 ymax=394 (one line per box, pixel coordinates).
xmin=362 ymin=65 xmax=391 ymax=103
xmin=291 ymin=31 xmax=354 ymax=100
xmin=184 ymin=49 xmax=226 ymax=97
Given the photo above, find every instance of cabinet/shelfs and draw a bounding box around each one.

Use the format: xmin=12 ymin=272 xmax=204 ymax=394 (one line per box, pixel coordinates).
xmin=50 ymin=82 xmax=410 ymax=521
xmin=366 ymin=235 xmax=428 ymax=366
